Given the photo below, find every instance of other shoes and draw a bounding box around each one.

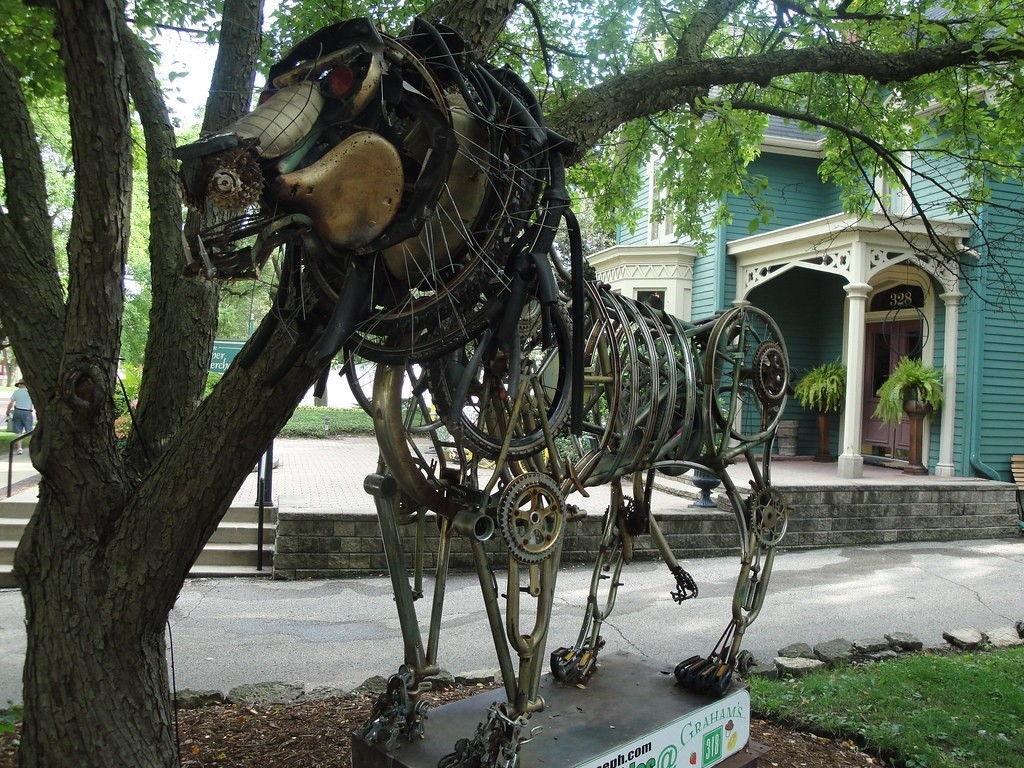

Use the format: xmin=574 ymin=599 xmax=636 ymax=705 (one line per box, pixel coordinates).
xmin=17 ymin=449 xmax=23 ymax=455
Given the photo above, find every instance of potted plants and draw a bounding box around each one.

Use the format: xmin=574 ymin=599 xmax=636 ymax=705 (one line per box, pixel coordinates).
xmin=793 ymin=356 xmax=847 ymax=464
xmin=686 ymin=398 xmax=737 ymax=508
xmin=869 ymin=355 xmax=952 ymax=475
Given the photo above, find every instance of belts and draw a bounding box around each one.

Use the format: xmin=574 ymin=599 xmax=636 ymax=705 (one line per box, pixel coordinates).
xmin=16 ymin=408 xmax=32 ymax=411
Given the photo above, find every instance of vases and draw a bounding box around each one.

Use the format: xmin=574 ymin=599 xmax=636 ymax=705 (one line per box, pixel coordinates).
xmin=776 ymin=419 xmax=799 ymax=457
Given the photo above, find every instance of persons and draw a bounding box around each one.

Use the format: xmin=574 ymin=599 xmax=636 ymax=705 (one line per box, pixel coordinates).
xmin=6 ymin=379 xmax=34 ymax=455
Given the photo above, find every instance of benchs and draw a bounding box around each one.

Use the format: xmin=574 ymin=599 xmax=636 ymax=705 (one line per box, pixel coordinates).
xmin=1010 ymin=454 xmax=1024 ymax=522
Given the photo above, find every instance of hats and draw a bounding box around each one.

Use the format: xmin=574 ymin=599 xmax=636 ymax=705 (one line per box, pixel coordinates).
xmin=15 ymin=379 xmax=25 ymax=387
